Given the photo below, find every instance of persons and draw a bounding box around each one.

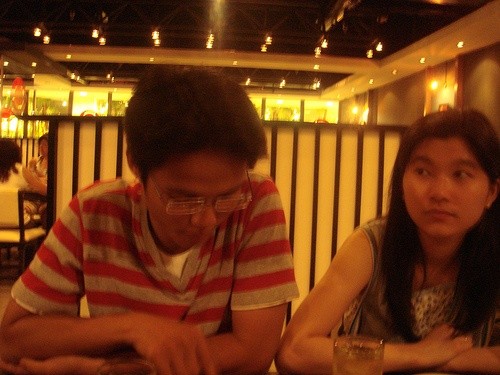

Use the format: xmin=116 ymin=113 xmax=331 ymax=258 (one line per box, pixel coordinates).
xmin=273 ymin=106 xmax=500 ymax=374
xmin=0 ymin=68 xmax=301 ymax=375
xmin=0 ymin=137 xmax=47 ymax=274
xmin=23 ymin=131 xmax=48 ymax=230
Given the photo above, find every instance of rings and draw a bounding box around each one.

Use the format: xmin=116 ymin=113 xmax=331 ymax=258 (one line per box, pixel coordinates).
xmin=464 ymin=336 xmax=467 ymax=343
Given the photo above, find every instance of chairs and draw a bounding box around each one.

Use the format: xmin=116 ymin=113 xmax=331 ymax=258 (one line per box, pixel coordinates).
xmin=0 ymin=187 xmax=49 ymax=284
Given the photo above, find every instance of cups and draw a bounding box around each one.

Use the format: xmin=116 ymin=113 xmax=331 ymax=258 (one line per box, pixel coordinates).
xmin=333 ymin=336 xmax=385 ymax=375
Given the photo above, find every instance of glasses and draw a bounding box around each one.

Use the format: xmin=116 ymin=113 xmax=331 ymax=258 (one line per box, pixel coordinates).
xmin=148 ymin=165 xmax=252 ymax=216
